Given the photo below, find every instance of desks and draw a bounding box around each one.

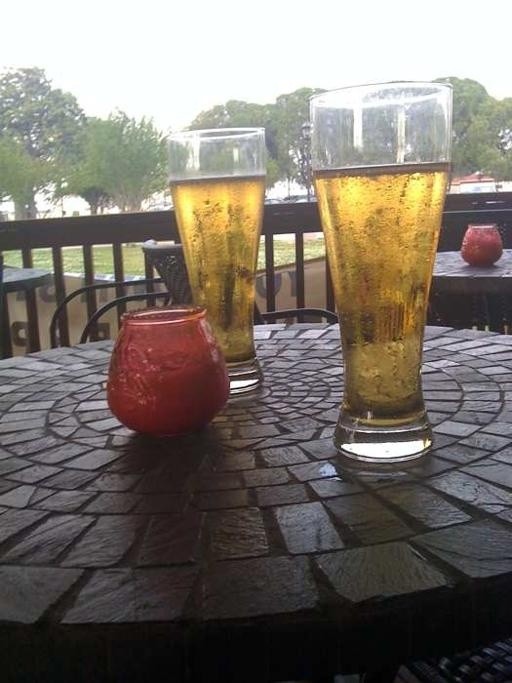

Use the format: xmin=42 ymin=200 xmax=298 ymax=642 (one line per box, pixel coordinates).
xmin=2 ymin=319 xmax=510 ymax=660
xmin=414 ymin=247 xmax=511 ymax=333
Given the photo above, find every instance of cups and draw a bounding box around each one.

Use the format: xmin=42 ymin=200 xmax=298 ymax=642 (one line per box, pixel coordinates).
xmin=305 ymin=80 xmax=455 ymax=466
xmin=167 ymin=127 xmax=267 ymax=397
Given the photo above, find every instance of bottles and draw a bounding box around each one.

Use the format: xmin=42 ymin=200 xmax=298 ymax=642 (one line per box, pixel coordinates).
xmin=461 ymin=220 xmax=503 ymax=268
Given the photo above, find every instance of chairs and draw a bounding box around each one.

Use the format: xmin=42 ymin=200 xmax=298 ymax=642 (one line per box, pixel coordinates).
xmin=142 ymin=239 xmax=340 ymax=323
